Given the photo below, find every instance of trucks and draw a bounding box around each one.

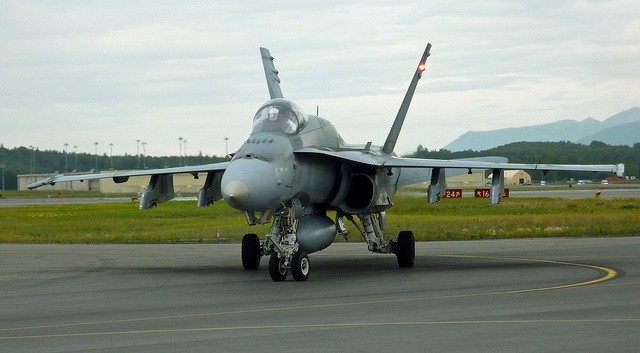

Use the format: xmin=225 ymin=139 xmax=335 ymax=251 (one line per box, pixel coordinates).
xmin=601 ymin=179 xmax=608 ymax=184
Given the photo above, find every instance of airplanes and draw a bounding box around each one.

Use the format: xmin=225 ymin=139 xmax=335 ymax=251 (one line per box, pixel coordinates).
xmin=27 ymin=43 xmax=618 ymax=280
